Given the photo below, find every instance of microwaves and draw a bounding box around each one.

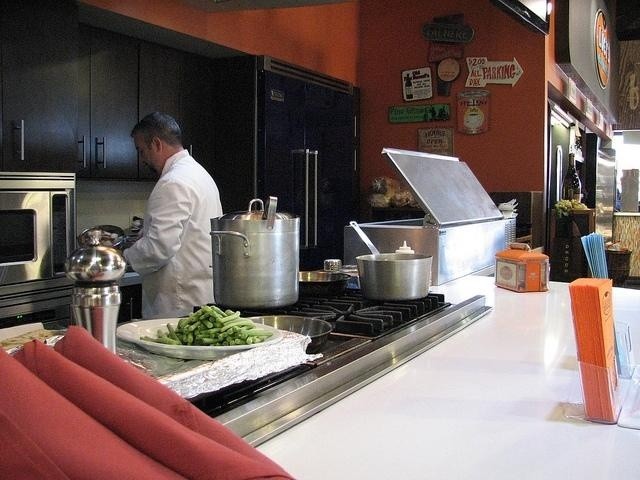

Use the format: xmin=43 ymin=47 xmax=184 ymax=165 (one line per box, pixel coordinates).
xmin=0 ymin=170 xmax=77 ymax=289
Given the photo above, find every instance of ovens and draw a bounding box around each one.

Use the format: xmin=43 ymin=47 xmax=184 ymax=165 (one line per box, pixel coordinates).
xmin=0 ymin=281 xmax=139 ymax=337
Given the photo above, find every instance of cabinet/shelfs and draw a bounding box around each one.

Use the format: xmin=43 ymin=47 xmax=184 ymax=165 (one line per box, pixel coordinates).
xmin=0 ymin=0 xmax=214 ymax=181
xmin=548 ymin=209 xmax=595 ymax=254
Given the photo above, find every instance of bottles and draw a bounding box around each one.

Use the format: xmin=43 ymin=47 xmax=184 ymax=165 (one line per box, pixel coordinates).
xmin=394 ymin=240 xmax=415 ymax=255
xmin=64 ymin=229 xmax=128 ymax=354
xmin=323 ymin=259 xmax=343 ymax=272
xmin=560 ymin=153 xmax=582 ymax=203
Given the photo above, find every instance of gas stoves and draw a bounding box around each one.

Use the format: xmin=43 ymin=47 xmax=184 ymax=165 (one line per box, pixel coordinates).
xmin=114 ymin=286 xmax=493 ymax=447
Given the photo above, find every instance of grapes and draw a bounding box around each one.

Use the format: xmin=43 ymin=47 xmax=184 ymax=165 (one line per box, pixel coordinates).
xmin=554 ymin=198 xmax=586 ymax=217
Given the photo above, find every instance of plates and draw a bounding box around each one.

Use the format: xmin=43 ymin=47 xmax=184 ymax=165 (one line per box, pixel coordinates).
xmin=114 ymin=318 xmax=285 ymax=360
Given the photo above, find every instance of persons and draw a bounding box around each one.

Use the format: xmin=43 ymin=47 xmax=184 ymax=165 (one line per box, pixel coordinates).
xmin=122 ymin=111 xmax=223 ymax=319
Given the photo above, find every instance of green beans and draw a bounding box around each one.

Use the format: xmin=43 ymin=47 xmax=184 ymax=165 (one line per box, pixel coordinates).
xmin=138 ymin=304 xmax=273 ymax=346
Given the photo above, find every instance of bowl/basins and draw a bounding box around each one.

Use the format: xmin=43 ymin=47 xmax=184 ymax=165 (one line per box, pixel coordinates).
xmin=245 ymin=315 xmax=333 ymax=353
xmin=298 ymin=270 xmax=352 ymax=299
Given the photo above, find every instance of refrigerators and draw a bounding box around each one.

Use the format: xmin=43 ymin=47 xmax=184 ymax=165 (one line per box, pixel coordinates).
xmin=197 ymin=55 xmax=361 ymax=270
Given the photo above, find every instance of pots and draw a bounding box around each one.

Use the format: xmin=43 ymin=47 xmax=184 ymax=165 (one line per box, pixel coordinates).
xmin=345 ymin=219 xmax=433 ymax=301
xmin=77 ymin=224 xmax=146 ymax=252
xmin=208 ymin=196 xmax=300 ymax=309
